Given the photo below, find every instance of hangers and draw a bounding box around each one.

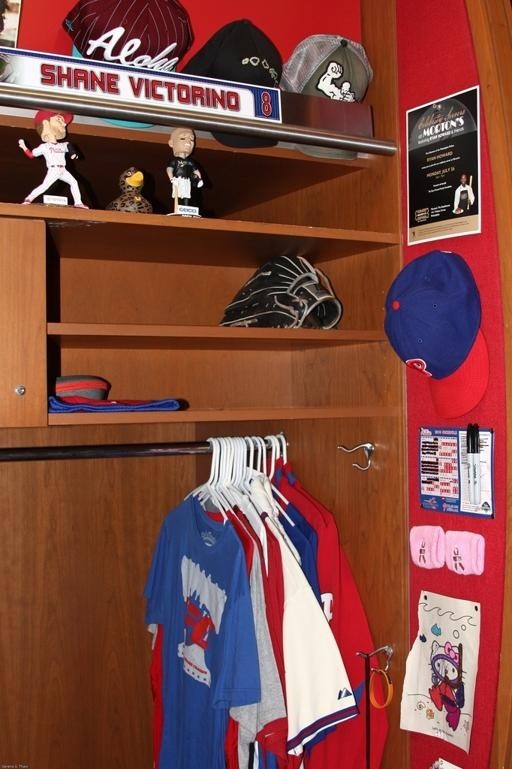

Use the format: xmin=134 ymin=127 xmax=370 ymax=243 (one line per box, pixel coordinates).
xmin=181 ymin=433 xmax=296 ymax=535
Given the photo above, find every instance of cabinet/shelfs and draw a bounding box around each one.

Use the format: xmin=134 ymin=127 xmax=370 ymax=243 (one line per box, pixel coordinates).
xmin=0 ymin=45 xmax=408 ymax=769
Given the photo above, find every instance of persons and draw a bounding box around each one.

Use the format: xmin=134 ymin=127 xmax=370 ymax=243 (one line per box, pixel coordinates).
xmin=166 ymin=127 xmax=205 ymax=218
xmin=451 ymin=176 xmax=474 ymax=213
xmin=18 ymin=110 xmax=89 ymax=209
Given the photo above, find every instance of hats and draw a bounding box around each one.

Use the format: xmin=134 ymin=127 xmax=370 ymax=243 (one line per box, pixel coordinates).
xmin=181 ymin=20 xmax=282 ymax=88
xmin=383 ymin=249 xmax=491 ymax=418
xmin=281 ymin=33 xmax=374 ymax=103
xmin=61 ymin=1 xmax=194 ymax=73
